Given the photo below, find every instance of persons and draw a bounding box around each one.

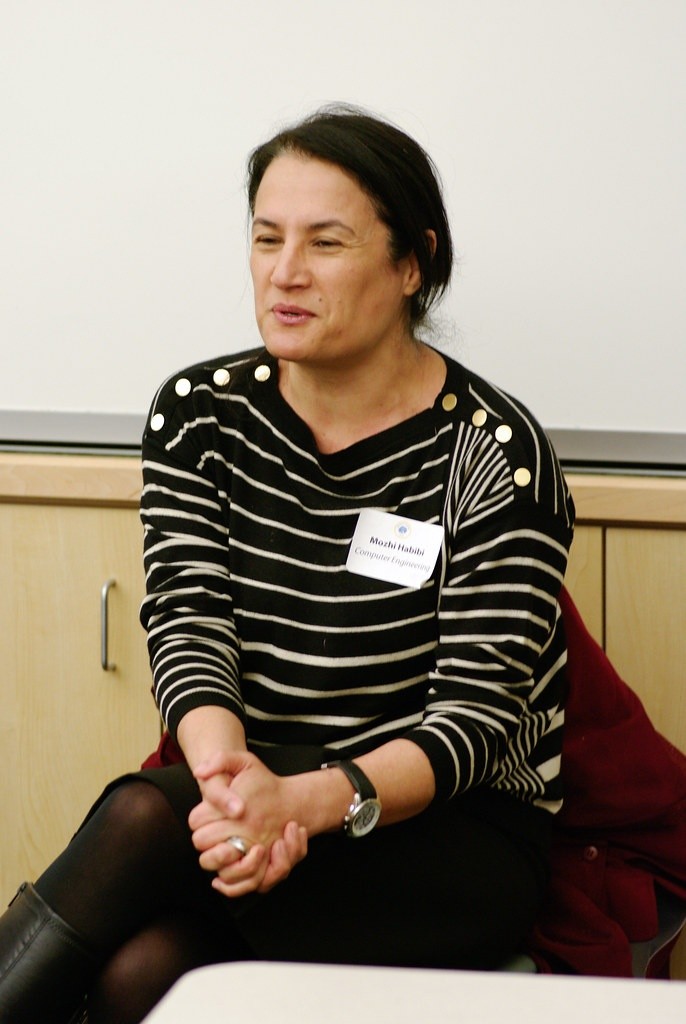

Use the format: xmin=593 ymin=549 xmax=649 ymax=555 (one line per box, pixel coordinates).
xmin=0 ymin=101 xmax=575 ymax=1024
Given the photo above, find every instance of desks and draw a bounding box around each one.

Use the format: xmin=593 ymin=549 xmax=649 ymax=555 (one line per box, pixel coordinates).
xmin=144 ymin=962 xmax=686 ymax=1024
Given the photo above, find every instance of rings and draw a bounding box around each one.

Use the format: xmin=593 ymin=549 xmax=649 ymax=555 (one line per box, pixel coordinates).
xmin=226 ymin=836 xmax=246 ymax=856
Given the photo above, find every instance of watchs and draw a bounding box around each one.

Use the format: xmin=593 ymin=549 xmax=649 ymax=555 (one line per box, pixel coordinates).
xmin=320 ymin=756 xmax=383 ymax=837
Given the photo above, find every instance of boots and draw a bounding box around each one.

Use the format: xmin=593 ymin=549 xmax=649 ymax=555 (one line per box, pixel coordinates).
xmin=0 ymin=883 xmax=110 ymax=1023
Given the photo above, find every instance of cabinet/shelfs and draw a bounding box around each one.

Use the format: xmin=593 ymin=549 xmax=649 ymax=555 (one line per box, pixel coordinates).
xmin=0 ymin=410 xmax=686 ymax=921
